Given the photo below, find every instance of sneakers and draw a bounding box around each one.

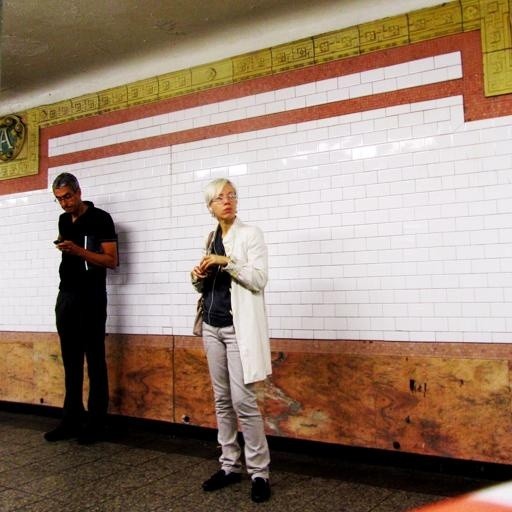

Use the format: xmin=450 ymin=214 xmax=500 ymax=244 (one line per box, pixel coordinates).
xmin=200 ymin=465 xmax=241 ymax=492
xmin=249 ymin=472 xmax=272 ymax=504
xmin=43 ymin=413 xmax=109 ymax=447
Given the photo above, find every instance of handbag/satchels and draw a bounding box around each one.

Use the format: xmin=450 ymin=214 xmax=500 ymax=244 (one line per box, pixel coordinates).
xmin=193 ymin=297 xmax=204 ymax=337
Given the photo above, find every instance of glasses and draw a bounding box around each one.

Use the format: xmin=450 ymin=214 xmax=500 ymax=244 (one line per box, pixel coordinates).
xmin=211 ymin=193 xmax=239 ymax=203
xmin=52 ymin=194 xmax=76 ymax=202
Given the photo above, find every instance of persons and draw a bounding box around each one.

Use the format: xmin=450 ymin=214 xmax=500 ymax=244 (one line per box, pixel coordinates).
xmin=189 ymin=178 xmax=272 ymax=502
xmin=45 ymin=173 xmax=118 ymax=444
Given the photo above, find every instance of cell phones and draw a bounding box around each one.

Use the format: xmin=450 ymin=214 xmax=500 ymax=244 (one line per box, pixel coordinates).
xmin=53 ymin=240 xmax=61 ymax=245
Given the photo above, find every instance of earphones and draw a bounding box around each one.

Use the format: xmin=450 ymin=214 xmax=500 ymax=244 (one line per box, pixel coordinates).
xmin=209 ymin=207 xmax=213 ymax=212
